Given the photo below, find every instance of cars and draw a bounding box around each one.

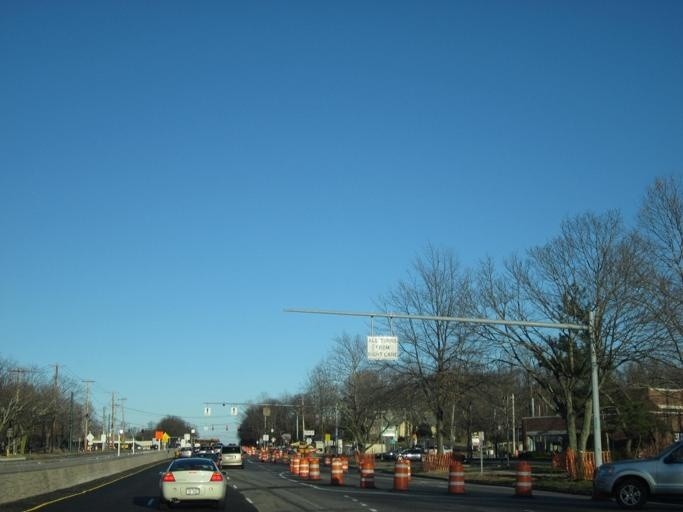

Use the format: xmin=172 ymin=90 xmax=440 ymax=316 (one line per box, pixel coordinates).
xmin=178 ymin=442 xmax=247 ymax=470
xmin=384 ymin=444 xmax=454 ymax=461
xmin=158 ymin=457 xmax=228 ymax=506
xmin=592 ymin=440 xmax=683 ymax=512
